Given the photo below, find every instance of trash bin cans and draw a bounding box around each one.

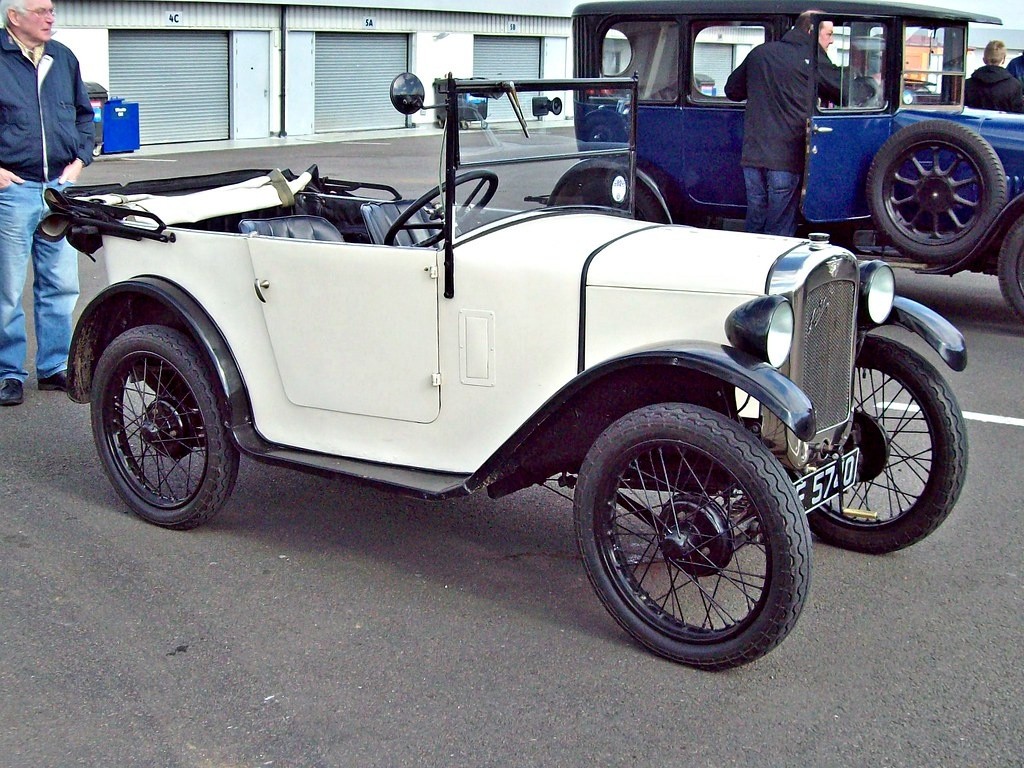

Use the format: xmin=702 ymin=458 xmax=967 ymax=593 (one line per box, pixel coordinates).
xmin=432 ymin=77 xmax=488 ymax=130
xmin=102 ymin=97 xmax=140 ymax=154
xmin=694 ymin=73 xmax=715 ymax=97
xmin=83 ymin=81 xmax=108 ymax=157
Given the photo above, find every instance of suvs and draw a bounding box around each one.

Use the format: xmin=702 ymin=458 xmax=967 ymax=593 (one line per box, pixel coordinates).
xmin=523 ymin=0 xmax=1024 ymax=323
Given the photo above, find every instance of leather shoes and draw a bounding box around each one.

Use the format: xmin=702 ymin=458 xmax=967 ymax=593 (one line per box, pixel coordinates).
xmin=0 ymin=378 xmax=24 ymax=405
xmin=38 ymin=369 xmax=67 ymax=392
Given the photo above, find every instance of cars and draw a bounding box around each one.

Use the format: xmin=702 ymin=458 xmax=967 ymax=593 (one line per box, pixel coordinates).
xmin=38 ymin=69 xmax=972 ymax=673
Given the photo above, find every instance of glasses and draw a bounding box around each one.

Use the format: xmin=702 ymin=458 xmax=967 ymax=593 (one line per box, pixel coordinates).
xmin=26 ymin=8 xmax=56 ymax=17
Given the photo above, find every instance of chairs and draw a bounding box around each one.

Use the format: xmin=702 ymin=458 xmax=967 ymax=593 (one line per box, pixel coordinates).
xmin=239 ymin=215 xmax=344 ymax=244
xmin=360 ymin=199 xmax=445 ymax=250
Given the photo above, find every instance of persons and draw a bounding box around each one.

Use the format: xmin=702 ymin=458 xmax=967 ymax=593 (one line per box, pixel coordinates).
xmin=965 ymin=39 xmax=1024 ymax=115
xmin=723 ymin=9 xmax=878 ymax=240
xmin=0 ymin=0 xmax=95 ymax=405
